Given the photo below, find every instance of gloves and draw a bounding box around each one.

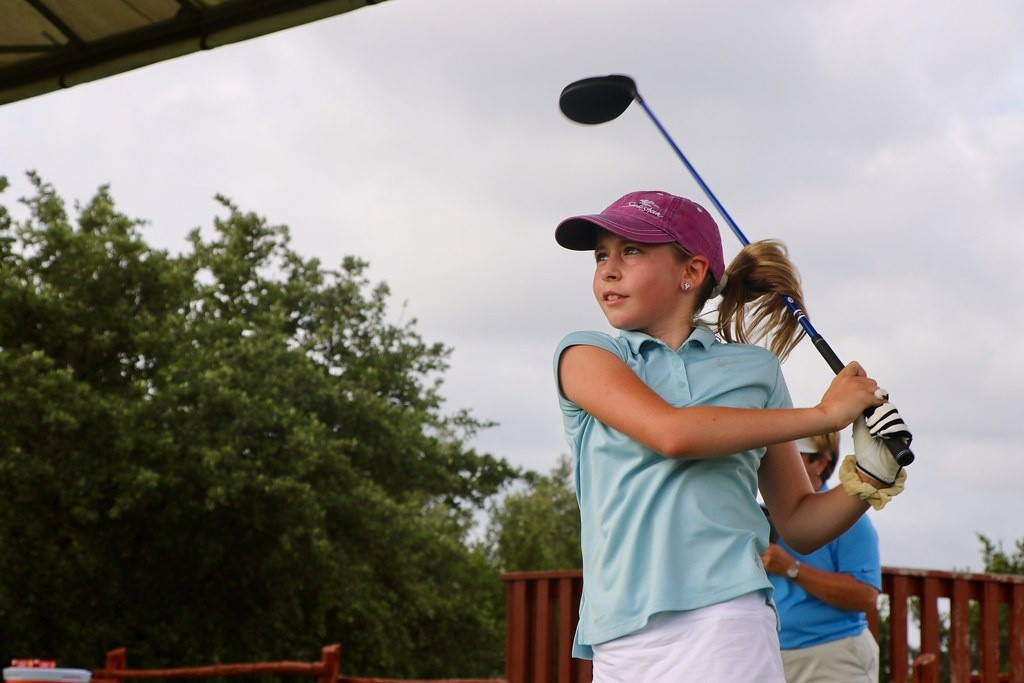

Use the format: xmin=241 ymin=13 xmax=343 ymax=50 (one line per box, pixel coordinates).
xmin=852 ymin=390 xmax=911 ymax=488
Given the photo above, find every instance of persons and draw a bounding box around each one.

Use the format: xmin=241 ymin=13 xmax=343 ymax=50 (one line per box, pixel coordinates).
xmin=554 ymin=190 xmax=913 ymax=683
xmin=761 ymin=432 xmax=882 ymax=683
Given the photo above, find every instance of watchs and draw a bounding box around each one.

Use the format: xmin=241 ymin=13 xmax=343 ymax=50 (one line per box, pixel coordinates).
xmin=787 ymin=560 xmax=800 ymax=578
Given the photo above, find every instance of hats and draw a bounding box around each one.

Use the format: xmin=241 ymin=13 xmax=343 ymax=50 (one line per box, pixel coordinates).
xmin=554 ymin=191 xmax=725 ymax=286
xmin=794 ymin=435 xmax=821 ymax=453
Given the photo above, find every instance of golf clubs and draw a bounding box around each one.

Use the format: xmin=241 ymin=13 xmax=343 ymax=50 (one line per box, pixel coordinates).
xmin=558 ymin=73 xmax=915 ymax=466
